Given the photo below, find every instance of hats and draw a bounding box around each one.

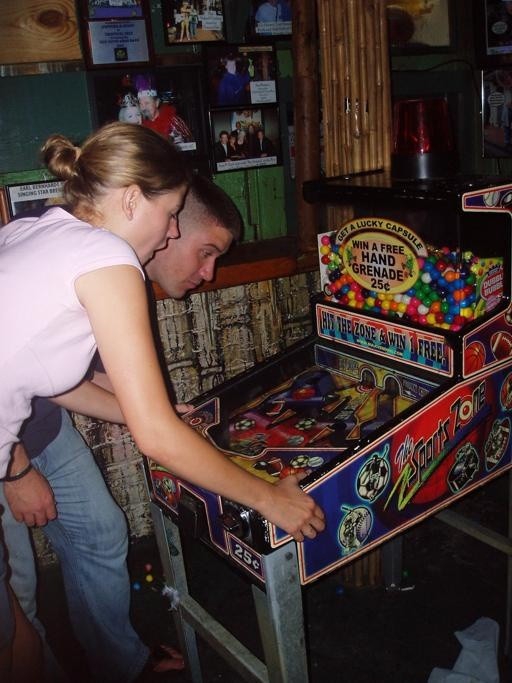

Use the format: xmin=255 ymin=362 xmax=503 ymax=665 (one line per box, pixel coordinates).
xmin=114 ymin=67 xmax=157 ymax=107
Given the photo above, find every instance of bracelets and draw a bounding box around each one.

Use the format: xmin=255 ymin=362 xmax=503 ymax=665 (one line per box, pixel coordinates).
xmin=2 ymin=462 xmax=35 ymax=482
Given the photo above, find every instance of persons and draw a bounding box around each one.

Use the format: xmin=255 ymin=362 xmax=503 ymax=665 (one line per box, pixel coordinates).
xmin=214 ymin=120 xmax=275 ymax=163
xmin=0 ymin=177 xmax=247 ymax=679
xmin=0 ymin=123 xmax=326 ymax=683
xmin=253 ymin=0 xmax=282 ymax=36
xmin=116 ymin=76 xmax=195 ymax=160
xmin=214 ymin=55 xmax=250 ymax=99
xmin=178 ymin=2 xmax=198 ymax=42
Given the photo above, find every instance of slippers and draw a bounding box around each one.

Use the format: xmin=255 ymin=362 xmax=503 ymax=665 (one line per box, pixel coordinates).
xmin=140 ymin=644 xmax=185 ymax=676
